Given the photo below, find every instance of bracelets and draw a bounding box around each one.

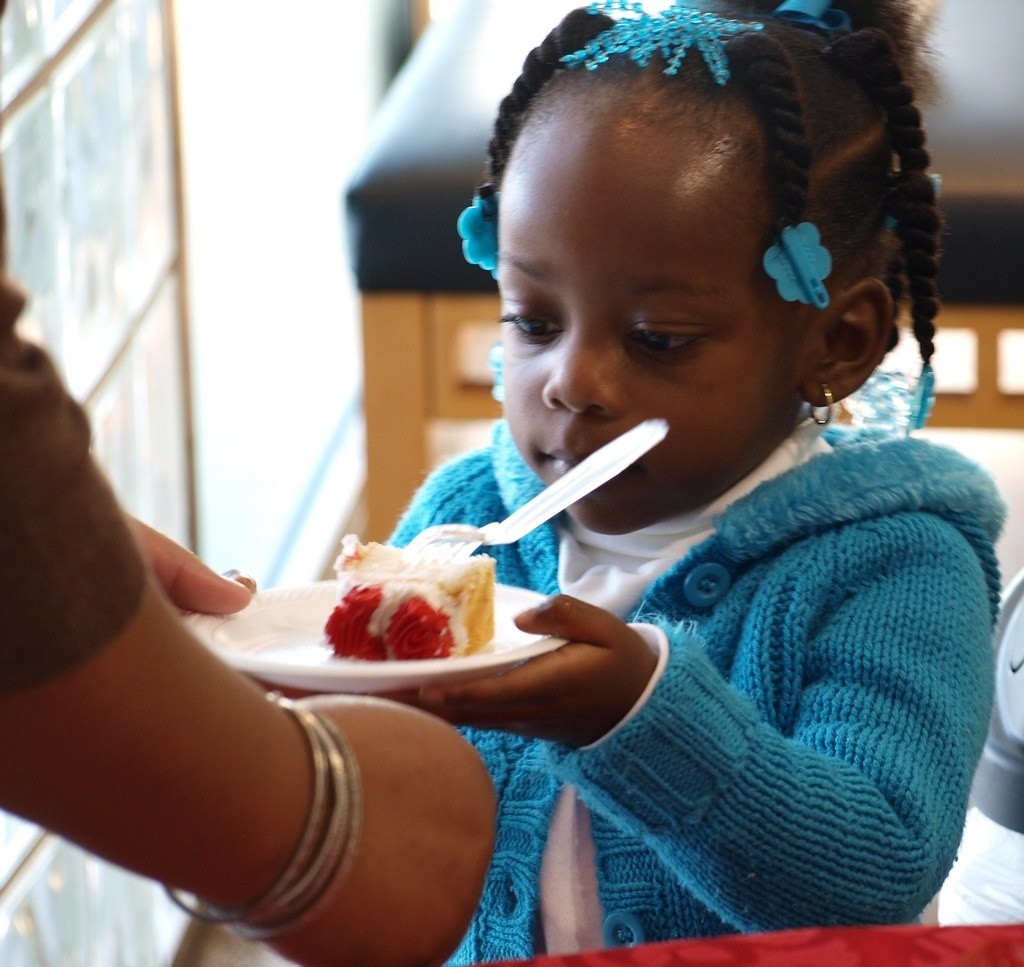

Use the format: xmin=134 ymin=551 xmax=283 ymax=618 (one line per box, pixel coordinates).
xmin=164 ymin=693 xmax=363 ymax=943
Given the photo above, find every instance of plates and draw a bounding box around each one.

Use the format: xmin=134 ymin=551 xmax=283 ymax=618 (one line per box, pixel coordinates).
xmin=183 ymin=580 xmax=567 ymax=692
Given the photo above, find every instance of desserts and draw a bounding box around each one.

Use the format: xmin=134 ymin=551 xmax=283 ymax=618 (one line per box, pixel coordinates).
xmin=323 ymin=533 xmax=496 ymax=660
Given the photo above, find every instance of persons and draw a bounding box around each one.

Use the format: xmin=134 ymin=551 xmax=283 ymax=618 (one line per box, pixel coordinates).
xmin=0 ymin=159 xmax=498 ymax=967
xmin=387 ymin=0 xmax=1006 ymax=967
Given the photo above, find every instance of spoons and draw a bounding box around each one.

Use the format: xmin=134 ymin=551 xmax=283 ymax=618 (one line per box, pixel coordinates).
xmin=406 ymin=418 xmax=669 ymax=558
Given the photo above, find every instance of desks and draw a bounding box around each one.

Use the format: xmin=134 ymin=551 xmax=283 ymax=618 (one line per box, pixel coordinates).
xmin=336 ymin=0 xmax=1024 ymax=554
